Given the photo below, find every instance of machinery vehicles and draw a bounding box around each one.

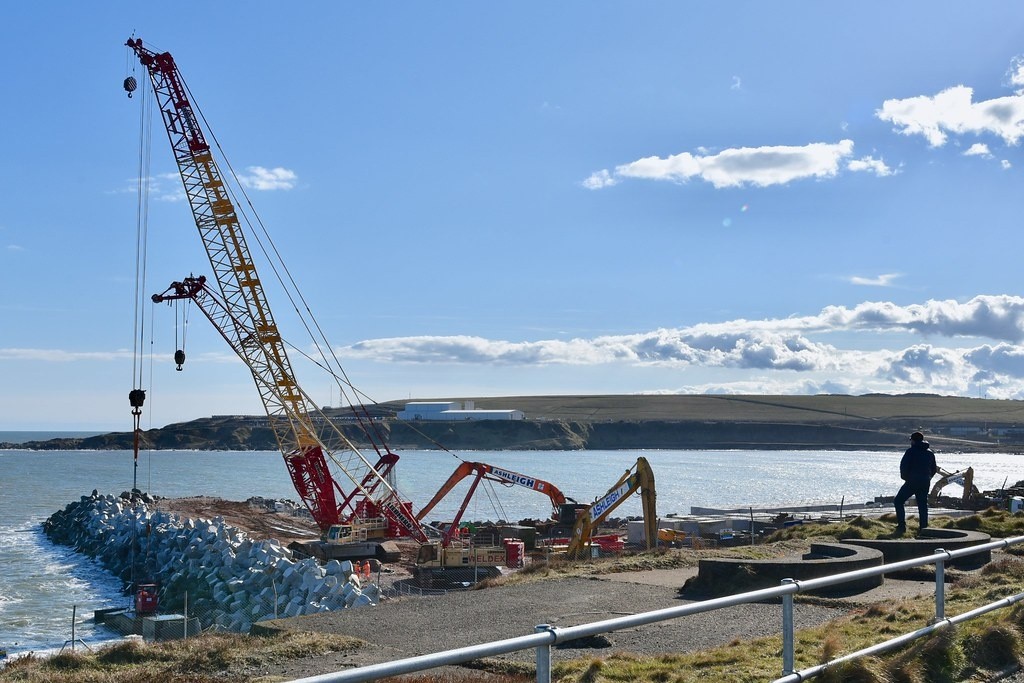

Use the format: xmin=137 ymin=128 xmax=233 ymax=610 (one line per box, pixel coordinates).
xmin=929 ymin=463 xmax=980 ymax=504
xmin=120 ymin=26 xmax=687 ymax=610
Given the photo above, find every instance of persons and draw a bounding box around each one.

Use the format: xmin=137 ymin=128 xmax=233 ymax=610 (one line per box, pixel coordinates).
xmin=363 ymin=561 xmax=370 ymax=582
xmin=355 ymin=561 xmax=361 ymax=578
xmin=893 ymin=431 xmax=936 ymax=534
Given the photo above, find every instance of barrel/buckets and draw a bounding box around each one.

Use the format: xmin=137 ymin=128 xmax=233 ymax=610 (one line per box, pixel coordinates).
xmin=503 ymin=537 xmax=525 ymax=569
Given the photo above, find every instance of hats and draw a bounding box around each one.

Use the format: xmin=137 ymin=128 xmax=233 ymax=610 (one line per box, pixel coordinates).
xmin=911 ymin=432 xmax=923 ymax=442
